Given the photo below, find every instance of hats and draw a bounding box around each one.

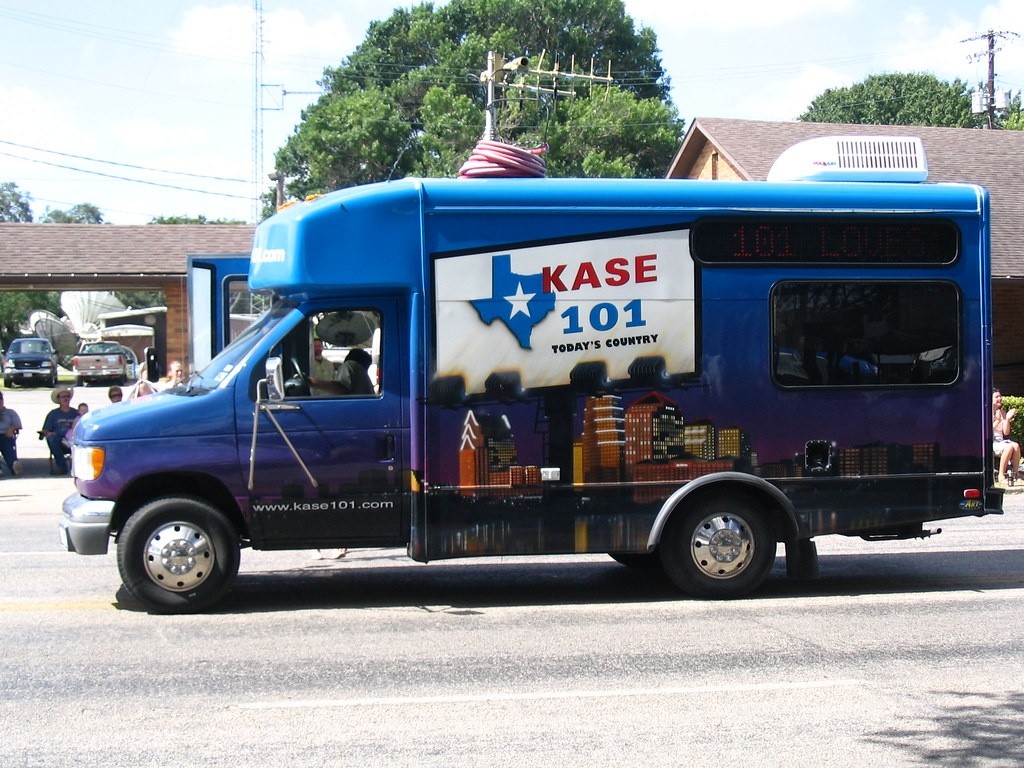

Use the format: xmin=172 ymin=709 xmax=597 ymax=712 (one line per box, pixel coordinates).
xmin=51 ymin=386 xmax=74 ymax=404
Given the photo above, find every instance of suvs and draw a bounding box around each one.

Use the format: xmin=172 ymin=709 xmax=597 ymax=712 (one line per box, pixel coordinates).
xmin=1 ymin=338 xmax=59 ymax=388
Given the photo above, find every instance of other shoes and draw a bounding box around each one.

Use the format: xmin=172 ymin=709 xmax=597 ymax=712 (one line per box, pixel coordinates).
xmin=998 ymin=474 xmax=1008 ymax=486
xmin=13 ymin=461 xmax=23 ymax=476
xmin=1014 ymin=478 xmax=1024 ymax=486
xmin=50 ymin=467 xmax=67 ymax=475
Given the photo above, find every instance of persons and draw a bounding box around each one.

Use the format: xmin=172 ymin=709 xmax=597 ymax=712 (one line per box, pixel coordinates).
xmin=992 ymin=387 xmax=1020 ymax=486
xmin=108 ymin=386 xmax=122 ymax=403
xmin=43 ymin=385 xmax=80 ymax=475
xmin=293 ymin=348 xmax=374 ymax=396
xmin=61 ymin=403 xmax=89 ymax=448
xmin=310 ymin=338 xmax=334 ymax=395
xmin=163 ymin=361 xmax=184 ymax=389
xmin=137 ymin=362 xmax=160 ymax=399
xmin=0 ymin=391 xmax=22 ymax=476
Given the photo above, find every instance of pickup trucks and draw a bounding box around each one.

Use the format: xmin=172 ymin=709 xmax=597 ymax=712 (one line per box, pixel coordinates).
xmin=74 ymin=341 xmax=128 ymax=387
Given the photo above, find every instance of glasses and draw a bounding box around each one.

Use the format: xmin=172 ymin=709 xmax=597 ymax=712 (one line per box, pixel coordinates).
xmin=110 ymin=394 xmax=121 ymax=397
xmin=60 ymin=395 xmax=70 ymax=398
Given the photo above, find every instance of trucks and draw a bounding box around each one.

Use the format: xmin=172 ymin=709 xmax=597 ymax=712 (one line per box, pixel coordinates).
xmin=62 ymin=136 xmax=1005 ymax=615
